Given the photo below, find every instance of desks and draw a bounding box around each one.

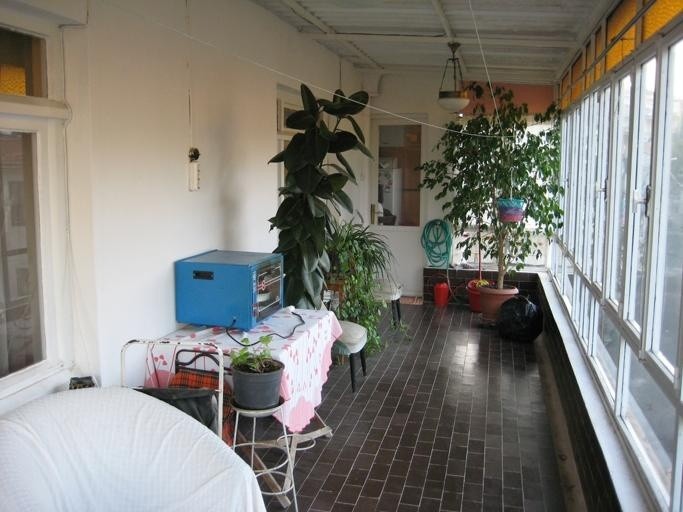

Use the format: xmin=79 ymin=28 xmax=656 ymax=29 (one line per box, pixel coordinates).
xmin=146 ymin=309 xmax=342 ymax=508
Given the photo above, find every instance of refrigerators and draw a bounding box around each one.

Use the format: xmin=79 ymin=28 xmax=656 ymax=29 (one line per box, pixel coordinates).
xmin=378 ymin=169 xmax=401 ymax=226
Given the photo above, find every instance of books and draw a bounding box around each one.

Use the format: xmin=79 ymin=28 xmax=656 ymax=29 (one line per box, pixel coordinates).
xmin=380 ymin=162 xmax=389 ymax=168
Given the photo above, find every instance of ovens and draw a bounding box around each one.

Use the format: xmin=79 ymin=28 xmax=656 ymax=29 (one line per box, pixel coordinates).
xmin=173 ymin=248 xmax=284 ymax=333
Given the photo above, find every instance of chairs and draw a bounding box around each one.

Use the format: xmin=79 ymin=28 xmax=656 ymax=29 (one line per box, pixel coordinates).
xmin=0 ymin=387 xmax=266 ymax=512
xmin=121 ymin=338 xmax=223 ymax=440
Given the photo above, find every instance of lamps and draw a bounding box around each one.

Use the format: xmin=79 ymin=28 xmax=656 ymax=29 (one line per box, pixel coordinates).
xmin=438 ymin=42 xmax=470 ymax=113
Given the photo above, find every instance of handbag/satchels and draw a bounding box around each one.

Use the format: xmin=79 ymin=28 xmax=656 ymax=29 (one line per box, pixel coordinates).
xmin=135 ymin=385 xmax=214 ymax=427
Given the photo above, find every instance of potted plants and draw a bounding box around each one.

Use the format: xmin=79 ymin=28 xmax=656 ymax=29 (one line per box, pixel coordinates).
xmin=229 ymin=335 xmax=285 ymax=408
xmin=416 ymin=80 xmax=565 ymax=317
xmin=324 ymin=211 xmax=415 ymax=357
xmin=452 ymin=181 xmax=496 ymax=312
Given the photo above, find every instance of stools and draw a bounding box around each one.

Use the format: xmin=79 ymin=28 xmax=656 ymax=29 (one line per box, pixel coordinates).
xmin=378 ymin=284 xmax=404 ymax=329
xmin=335 ymin=321 xmax=368 ymax=392
